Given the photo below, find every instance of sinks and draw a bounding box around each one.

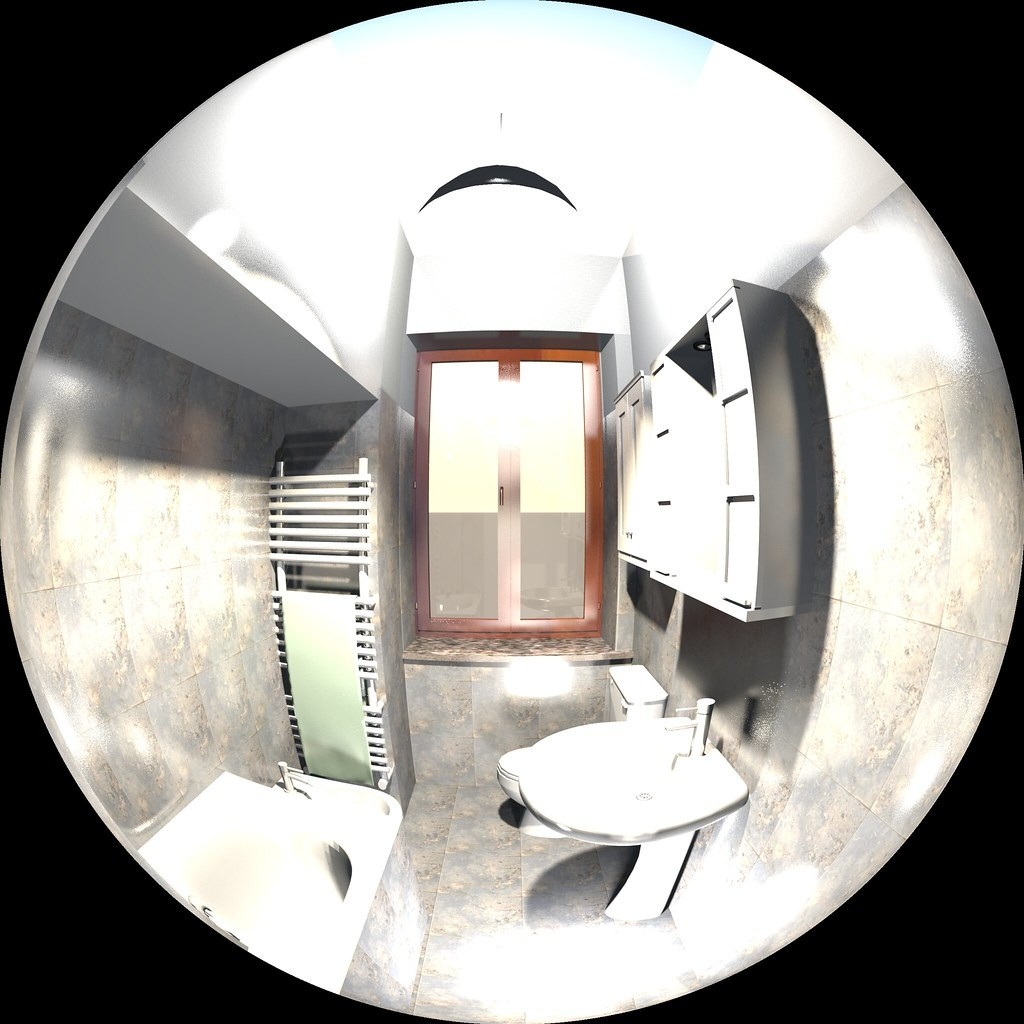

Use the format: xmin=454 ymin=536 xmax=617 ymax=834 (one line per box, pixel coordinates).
xmin=519 ymin=717 xmax=750 ymax=847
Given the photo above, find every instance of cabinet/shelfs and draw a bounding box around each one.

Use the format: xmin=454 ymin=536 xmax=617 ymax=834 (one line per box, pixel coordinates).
xmin=649 ymin=278 xmax=816 ymax=623
xmin=612 ymin=368 xmax=649 ymax=572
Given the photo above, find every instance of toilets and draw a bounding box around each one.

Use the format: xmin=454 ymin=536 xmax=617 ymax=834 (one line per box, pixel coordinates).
xmin=495 ymin=664 xmax=670 ymax=837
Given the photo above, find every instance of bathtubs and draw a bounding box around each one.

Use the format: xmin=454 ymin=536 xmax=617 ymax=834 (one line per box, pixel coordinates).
xmin=138 ymin=763 xmax=427 ymax=1016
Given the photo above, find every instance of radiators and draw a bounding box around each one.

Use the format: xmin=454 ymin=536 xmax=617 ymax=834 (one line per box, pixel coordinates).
xmin=268 ymin=457 xmax=393 ymax=791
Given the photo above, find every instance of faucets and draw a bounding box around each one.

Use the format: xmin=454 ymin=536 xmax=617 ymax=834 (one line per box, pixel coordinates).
xmin=664 ymin=697 xmax=717 ymax=759
xmin=278 ymin=761 xmax=313 ymax=793
